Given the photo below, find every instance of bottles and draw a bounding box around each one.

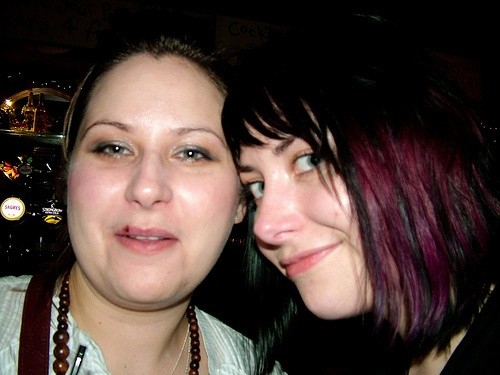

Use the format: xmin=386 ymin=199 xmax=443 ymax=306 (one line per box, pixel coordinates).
xmin=36 ymin=92 xmax=48 ymax=132
xmin=24 ymin=91 xmax=36 ymax=131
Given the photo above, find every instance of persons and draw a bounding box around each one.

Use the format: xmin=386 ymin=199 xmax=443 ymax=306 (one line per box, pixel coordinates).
xmin=223 ymin=12 xmax=500 ymax=375
xmin=0 ymin=34 xmax=288 ymax=375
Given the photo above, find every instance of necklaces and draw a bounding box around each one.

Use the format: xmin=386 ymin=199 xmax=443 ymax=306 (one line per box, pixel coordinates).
xmin=170 ymin=324 xmax=192 ymax=375
xmin=53 ymin=267 xmax=201 ymax=375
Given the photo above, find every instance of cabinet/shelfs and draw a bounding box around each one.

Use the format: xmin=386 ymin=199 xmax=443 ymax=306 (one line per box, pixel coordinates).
xmin=0 ymin=126 xmax=75 ymax=277
